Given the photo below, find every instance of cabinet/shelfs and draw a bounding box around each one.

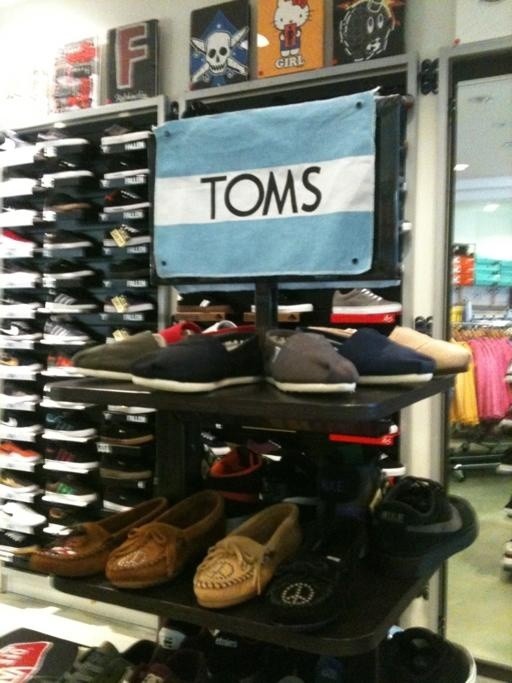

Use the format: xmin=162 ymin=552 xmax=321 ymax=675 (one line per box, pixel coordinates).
xmin=49 ymin=373 xmax=479 ymax=656
xmin=0 ymin=95 xmax=166 ymax=632
xmin=495 ymin=368 xmax=512 ymax=586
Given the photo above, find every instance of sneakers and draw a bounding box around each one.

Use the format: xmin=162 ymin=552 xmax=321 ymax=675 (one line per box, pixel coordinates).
xmin=103 ymin=188 xmax=151 ymax=214
xmin=0 ymin=470 xmax=41 ymax=495
xmin=0 ymin=502 xmax=46 ymax=528
xmin=36 ymin=129 xmax=91 ymax=149
xmin=0 ymin=319 xmax=42 ymax=340
xmin=4 ymin=387 xmax=41 ymax=406
xmin=45 ymin=479 xmax=97 ymax=502
xmin=191 ymin=430 xmax=233 ymax=456
xmin=105 ymin=489 xmax=226 ymax=590
xmin=99 ymin=411 xmax=154 ymax=445
xmin=207 ymin=632 xmax=265 ymax=683
xmin=264 ymin=449 xmax=321 ymax=505
xmin=100 ymin=454 xmax=152 ymax=480
xmin=40 ymin=159 xmax=96 ymax=188
xmin=373 ymin=417 xmax=400 ymax=433
xmin=103 ymin=154 xmax=150 ymax=180
xmin=295 ymin=327 xmax=435 ymax=386
xmin=332 ymin=288 xmax=402 ymax=315
xmin=101 ymin=120 xmax=153 ymax=146
xmin=42 ymin=259 xmax=96 ymax=280
xmin=109 ymin=259 xmax=153 ymax=278
xmin=2 ymin=411 xmax=42 ymax=434
xmin=3 ymin=229 xmax=37 ymax=250
xmin=44 ymin=443 xmax=99 ymax=469
xmin=68 ymin=330 xmax=162 ymax=381
xmin=370 ymin=476 xmax=476 ymax=580
xmin=177 ymin=292 xmax=235 ymax=312
xmin=307 ymin=325 xmax=470 ymax=375
xmin=366 ymin=449 xmax=406 ymax=475
xmin=2 ymin=262 xmax=41 ymax=282
xmin=0 ymin=531 xmax=43 ymax=554
xmin=250 ymin=291 xmax=314 ymax=314
xmin=43 ymin=229 xmax=94 ymax=250
xmin=44 ymin=289 xmax=99 ymax=310
xmin=43 ymin=317 xmax=90 ymax=341
xmin=43 ymin=413 xmax=97 ymax=437
xmin=3 ymin=205 xmax=39 ymax=218
xmin=381 ymin=628 xmax=477 ymax=682
xmin=268 ymin=504 xmax=376 ymax=635
xmin=160 ymin=320 xmax=255 ymax=345
xmin=201 ymin=319 xmax=238 ymax=334
xmin=1 ymin=441 xmax=44 ymax=462
xmin=120 ymin=649 xmax=171 ymax=683
xmin=48 ymin=507 xmax=101 ymax=536
xmin=103 ymin=488 xmax=145 ymax=513
xmin=210 ymin=440 xmax=281 ymax=479
xmin=0 ymin=292 xmax=44 ymax=310
xmin=159 ymin=619 xmax=200 ymax=651
xmin=0 ymin=350 xmax=41 ymax=371
xmin=202 ymin=447 xmax=279 ymax=534
xmin=142 ymin=636 xmax=211 ymax=683
xmin=104 ymin=223 xmax=152 ymax=247
xmin=194 ymin=503 xmax=303 ymax=609
xmin=56 ymin=640 xmax=160 ymax=683
xmin=46 ymin=193 xmax=93 ymax=213
xmin=262 ymin=330 xmax=359 ymax=394
xmin=131 ymin=332 xmax=265 ymax=393
xmin=47 ymin=350 xmax=75 ymax=371
xmin=30 ymin=498 xmax=172 ymax=578
xmin=103 ymin=293 xmax=154 ymax=313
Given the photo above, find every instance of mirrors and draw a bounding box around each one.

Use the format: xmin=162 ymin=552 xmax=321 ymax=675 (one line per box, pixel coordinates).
xmin=437 ymin=47 xmax=511 ymax=683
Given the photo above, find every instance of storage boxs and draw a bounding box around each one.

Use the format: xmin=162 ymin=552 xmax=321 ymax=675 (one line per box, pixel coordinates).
xmin=450 ymin=243 xmax=512 ymax=286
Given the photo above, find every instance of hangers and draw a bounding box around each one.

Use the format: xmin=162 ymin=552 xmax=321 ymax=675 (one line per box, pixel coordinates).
xmin=451 ymin=321 xmax=512 ymax=340
